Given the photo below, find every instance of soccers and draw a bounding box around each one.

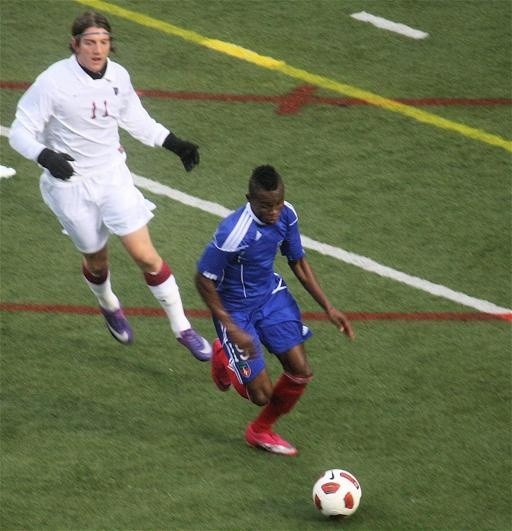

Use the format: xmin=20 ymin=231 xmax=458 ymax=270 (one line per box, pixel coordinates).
xmin=313 ymin=469 xmax=361 ymax=519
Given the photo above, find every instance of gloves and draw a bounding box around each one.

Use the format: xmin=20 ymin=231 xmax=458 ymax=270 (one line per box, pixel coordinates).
xmin=36 ymin=147 xmax=75 ymax=182
xmin=162 ymin=131 xmax=202 ymax=173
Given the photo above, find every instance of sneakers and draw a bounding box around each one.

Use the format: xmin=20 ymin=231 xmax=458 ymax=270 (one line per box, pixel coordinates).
xmin=244 ymin=422 xmax=298 ymax=459
xmin=209 ymin=335 xmax=233 ymax=392
xmin=97 ymin=299 xmax=135 ymax=346
xmin=175 ymin=326 xmax=214 ymax=361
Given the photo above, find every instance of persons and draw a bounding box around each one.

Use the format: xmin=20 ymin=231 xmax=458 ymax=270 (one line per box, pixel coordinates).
xmin=194 ymin=165 xmax=353 ymax=455
xmin=8 ymin=11 xmax=213 ymax=362
